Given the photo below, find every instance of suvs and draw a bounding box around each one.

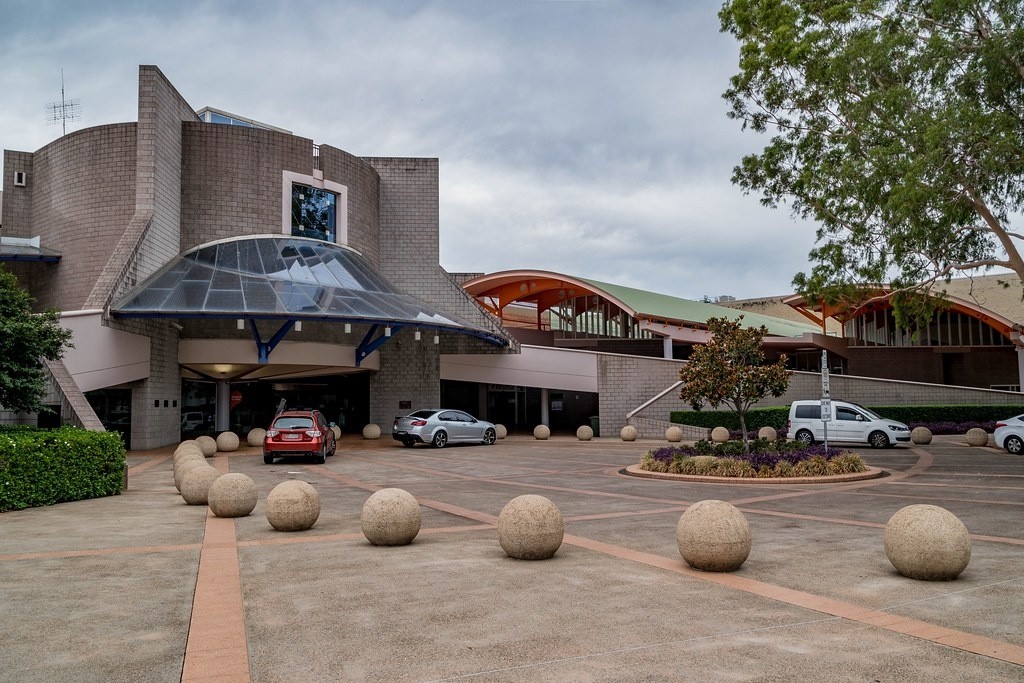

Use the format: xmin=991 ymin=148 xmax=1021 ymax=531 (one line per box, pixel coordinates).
xmin=263 ymin=409 xmax=336 ymax=464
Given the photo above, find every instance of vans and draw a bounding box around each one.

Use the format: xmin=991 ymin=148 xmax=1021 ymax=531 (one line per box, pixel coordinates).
xmin=181 ymin=411 xmax=210 ymax=433
xmin=785 ymin=399 xmax=912 ymax=449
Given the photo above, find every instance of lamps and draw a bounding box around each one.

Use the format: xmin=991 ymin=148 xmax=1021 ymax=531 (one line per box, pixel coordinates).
xmin=385 ymin=324 xmax=391 ymax=336
xmin=344 ymin=322 xmax=351 ymax=333
xmin=295 ymin=320 xmax=302 ymax=332
xmin=237 ymin=319 xmax=244 ymax=329
xmin=433 ymin=329 xmax=439 ymax=344
xmin=415 ymin=327 xmax=420 ymax=340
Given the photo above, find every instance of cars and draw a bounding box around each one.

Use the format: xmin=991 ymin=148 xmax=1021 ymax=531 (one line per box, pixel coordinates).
xmin=392 ymin=408 xmax=498 ymax=448
xmin=993 ymin=414 xmax=1024 ymax=455
